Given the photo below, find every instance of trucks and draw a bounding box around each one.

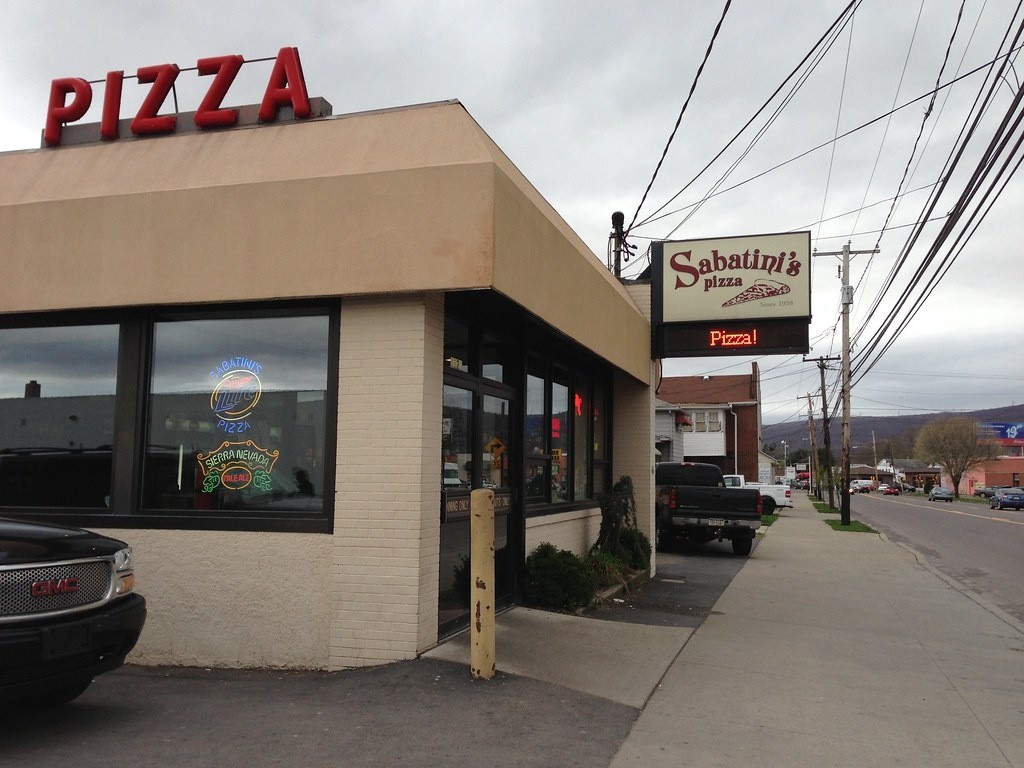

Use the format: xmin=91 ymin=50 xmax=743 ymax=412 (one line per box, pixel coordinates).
xmin=850 ymin=480 xmax=872 ymax=488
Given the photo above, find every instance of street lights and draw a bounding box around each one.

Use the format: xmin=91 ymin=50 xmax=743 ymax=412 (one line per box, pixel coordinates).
xmin=853 ymin=446 xmax=878 ymax=492
xmin=781 ymin=441 xmax=786 ymax=474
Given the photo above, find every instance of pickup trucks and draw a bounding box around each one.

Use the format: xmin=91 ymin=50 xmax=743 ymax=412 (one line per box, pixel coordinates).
xmin=656 ymin=462 xmax=762 ymax=555
xmin=718 ymin=474 xmax=793 ymax=515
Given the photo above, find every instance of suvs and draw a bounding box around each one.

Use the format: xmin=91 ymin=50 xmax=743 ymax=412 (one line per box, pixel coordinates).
xmin=0 ymin=519 xmax=146 ymax=702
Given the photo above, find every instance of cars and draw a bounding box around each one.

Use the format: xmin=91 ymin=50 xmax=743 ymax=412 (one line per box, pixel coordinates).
xmin=840 ymin=482 xmax=916 ymax=496
xmin=928 ymin=487 xmax=953 ymax=502
xmin=775 ymin=476 xmax=810 ymax=490
xmin=974 ymin=486 xmax=1024 ymax=510
